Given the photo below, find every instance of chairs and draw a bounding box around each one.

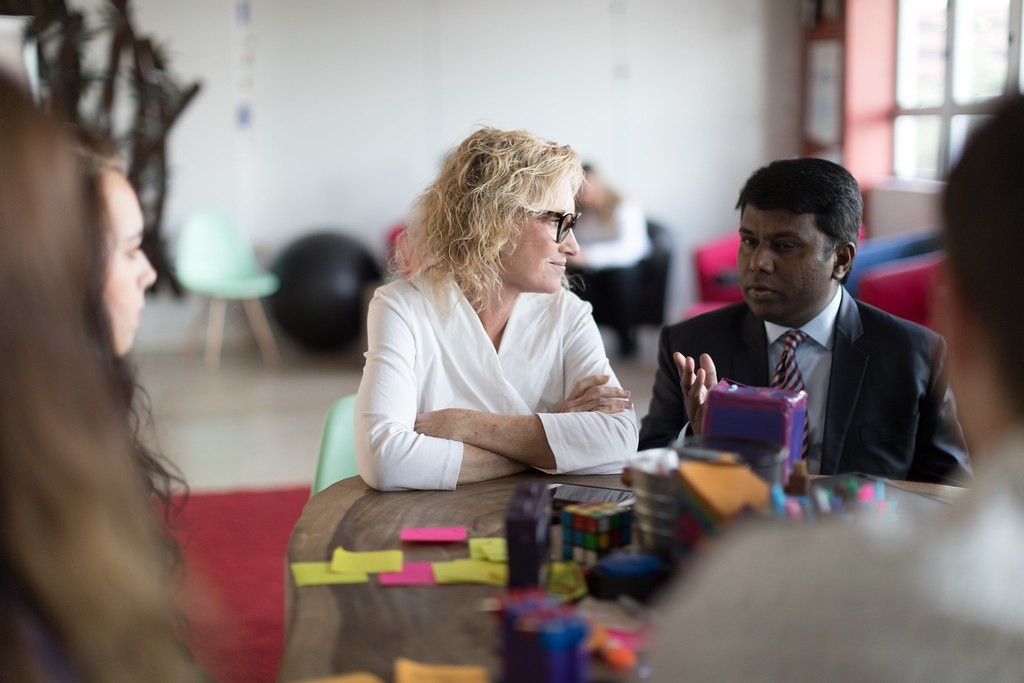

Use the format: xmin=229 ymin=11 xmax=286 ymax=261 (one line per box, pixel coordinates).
xmin=174 ymin=209 xmax=283 ymax=376
xmin=594 ymin=223 xmax=676 ymax=361
xmin=683 ymin=230 xmax=973 ymax=372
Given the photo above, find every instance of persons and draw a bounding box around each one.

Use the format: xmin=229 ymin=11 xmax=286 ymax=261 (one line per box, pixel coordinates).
xmin=638 ymin=157 xmax=975 ymax=490
xmin=353 ymin=127 xmax=649 ymax=489
xmin=639 ymin=89 xmax=1024 ymax=683
xmin=562 ymin=163 xmax=656 ymax=358
xmin=0 ymin=126 xmax=203 ymax=683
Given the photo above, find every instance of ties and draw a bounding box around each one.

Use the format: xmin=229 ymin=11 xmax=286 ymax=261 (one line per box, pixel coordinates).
xmin=770 ymin=330 xmax=812 ymax=467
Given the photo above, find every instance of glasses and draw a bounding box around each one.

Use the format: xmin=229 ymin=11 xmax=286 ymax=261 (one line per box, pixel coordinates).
xmin=514 ymin=204 xmax=584 ymax=245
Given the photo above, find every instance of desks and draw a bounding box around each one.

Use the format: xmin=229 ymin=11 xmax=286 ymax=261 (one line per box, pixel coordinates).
xmin=275 ymin=465 xmax=974 ymax=683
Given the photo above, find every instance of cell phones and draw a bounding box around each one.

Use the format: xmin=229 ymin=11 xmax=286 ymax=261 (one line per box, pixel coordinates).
xmin=547 ymin=482 xmax=632 ymax=507
